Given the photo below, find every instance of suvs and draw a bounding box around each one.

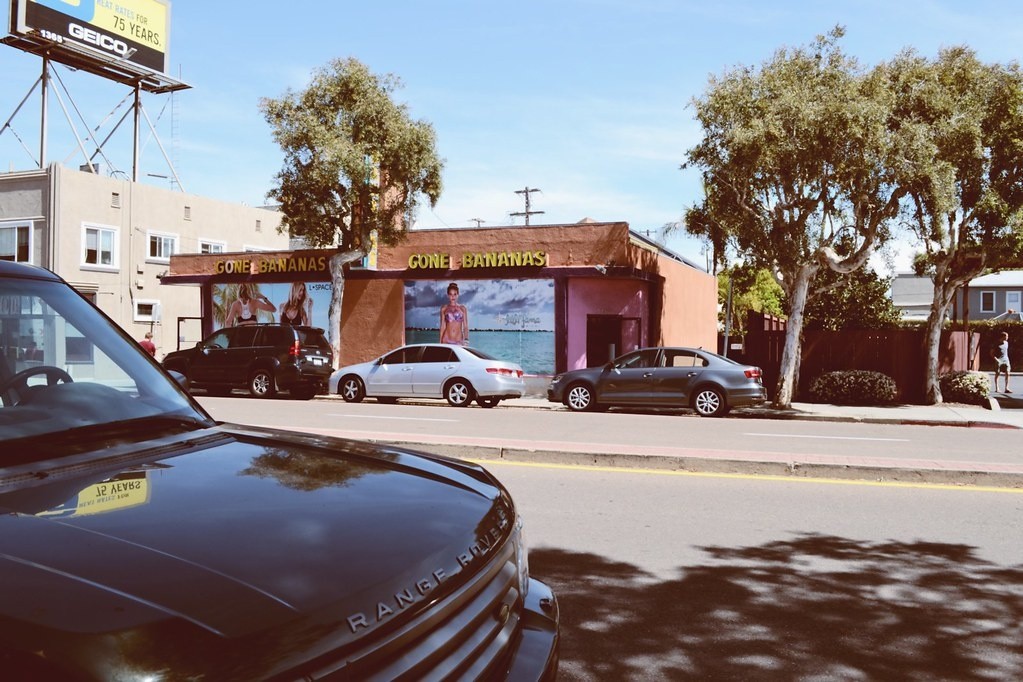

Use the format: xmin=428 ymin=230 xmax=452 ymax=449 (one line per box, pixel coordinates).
xmin=160 ymin=321 xmax=333 ymax=399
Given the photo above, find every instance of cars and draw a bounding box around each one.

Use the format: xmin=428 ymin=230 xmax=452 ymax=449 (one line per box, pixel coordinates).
xmin=546 ymin=346 xmax=768 ymax=419
xmin=331 ymin=341 xmax=524 ymax=409
xmin=0 ymin=256 xmax=567 ymax=682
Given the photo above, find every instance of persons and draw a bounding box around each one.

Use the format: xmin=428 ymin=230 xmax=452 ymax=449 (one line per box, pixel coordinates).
xmin=989 ymin=331 xmax=1012 ymax=394
xmin=225 ymin=283 xmax=276 ymax=327
xmin=137 ymin=333 xmax=156 ymax=358
xmin=278 ymin=280 xmax=315 ymax=328
xmin=439 ymin=281 xmax=470 ymax=348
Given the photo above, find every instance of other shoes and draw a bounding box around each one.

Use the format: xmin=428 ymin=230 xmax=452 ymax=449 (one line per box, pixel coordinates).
xmin=1005 ymin=390 xmax=1013 ymax=393
xmin=995 ymin=391 xmax=1000 ymax=393
